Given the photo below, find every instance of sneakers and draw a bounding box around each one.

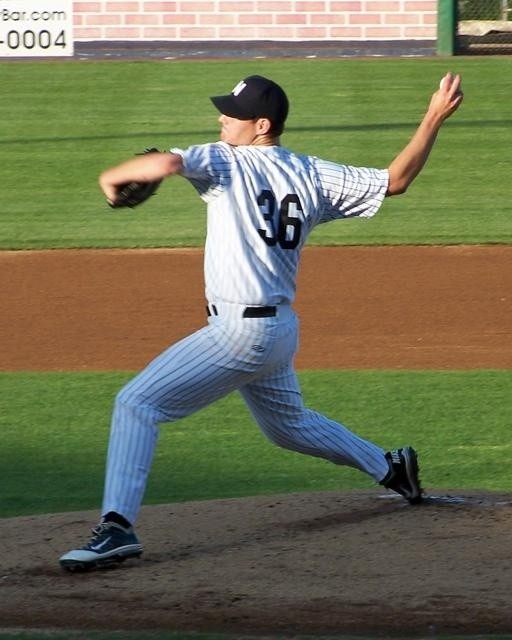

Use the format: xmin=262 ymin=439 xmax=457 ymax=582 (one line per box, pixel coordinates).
xmin=57 ymin=520 xmax=143 ymax=569
xmin=380 ymin=445 xmax=423 ymax=508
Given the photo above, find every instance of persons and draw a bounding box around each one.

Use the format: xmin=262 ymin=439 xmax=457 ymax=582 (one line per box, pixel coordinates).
xmin=57 ymin=71 xmax=465 ymax=569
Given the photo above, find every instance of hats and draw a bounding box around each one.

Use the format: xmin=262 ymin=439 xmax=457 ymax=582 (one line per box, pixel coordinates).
xmin=210 ymin=75 xmax=289 ymax=123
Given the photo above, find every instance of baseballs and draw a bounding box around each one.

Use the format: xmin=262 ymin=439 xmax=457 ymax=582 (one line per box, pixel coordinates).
xmin=440 ymin=74 xmax=461 ymax=93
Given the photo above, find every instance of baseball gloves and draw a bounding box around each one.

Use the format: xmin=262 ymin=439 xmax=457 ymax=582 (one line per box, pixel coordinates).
xmin=104 ymin=148 xmax=163 ymax=209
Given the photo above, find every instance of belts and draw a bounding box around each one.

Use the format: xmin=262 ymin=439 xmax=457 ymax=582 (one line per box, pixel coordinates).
xmin=205 ymin=303 xmax=276 ymax=319
